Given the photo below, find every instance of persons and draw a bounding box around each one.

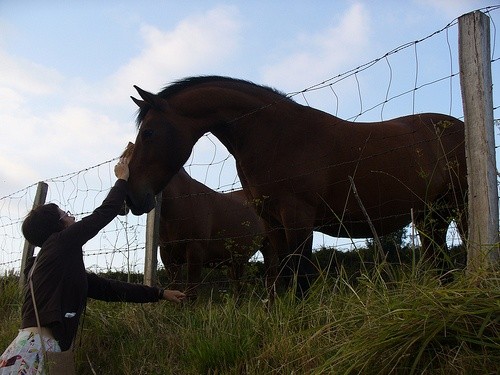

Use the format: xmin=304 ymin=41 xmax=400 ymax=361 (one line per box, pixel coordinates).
xmin=0 ymin=158 xmax=187 ymax=374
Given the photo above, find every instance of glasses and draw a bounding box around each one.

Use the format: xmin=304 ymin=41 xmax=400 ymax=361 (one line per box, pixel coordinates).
xmin=58 ymin=210 xmax=72 ymax=220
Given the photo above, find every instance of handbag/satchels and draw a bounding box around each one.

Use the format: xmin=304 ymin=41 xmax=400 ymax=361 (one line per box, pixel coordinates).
xmin=44 ymin=352 xmax=75 ymax=375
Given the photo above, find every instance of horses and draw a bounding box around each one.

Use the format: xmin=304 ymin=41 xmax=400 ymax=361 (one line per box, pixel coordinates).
xmin=124 ymin=143 xmax=280 ymax=310
xmin=125 ymin=76 xmax=468 ymax=311
